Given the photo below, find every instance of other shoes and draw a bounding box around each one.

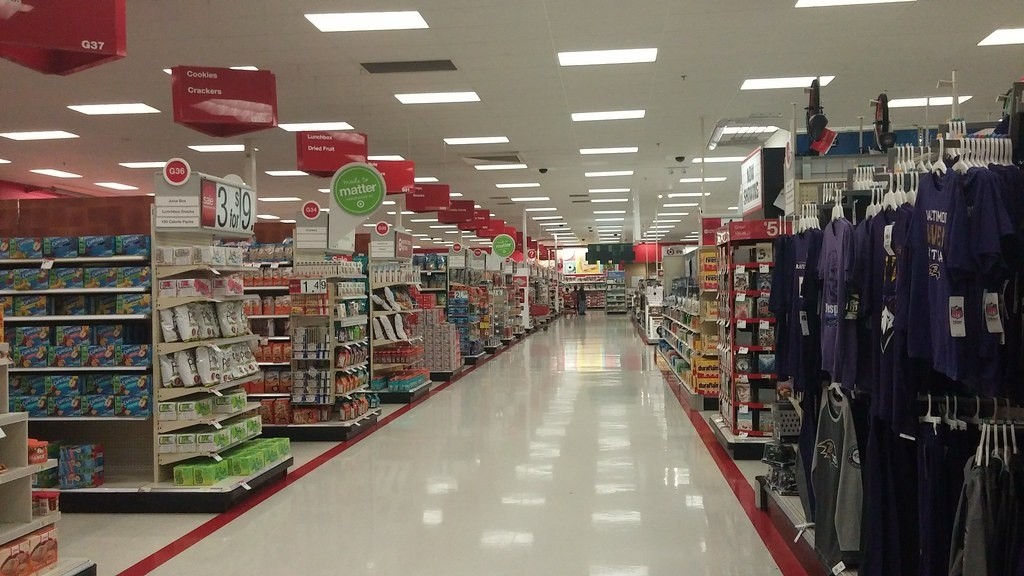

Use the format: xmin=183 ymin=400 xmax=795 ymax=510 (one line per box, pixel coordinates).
xmin=579 ymin=313 xmax=586 ymax=315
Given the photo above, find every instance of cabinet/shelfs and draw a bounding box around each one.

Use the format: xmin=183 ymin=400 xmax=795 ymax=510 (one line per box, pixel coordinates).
xmin=709 ymin=219 xmax=788 ymax=460
xmin=243 ymin=243 xmax=432 ymax=442
xmin=0 ymin=342 xmax=98 ymax=576
xmin=0 ymin=195 xmax=294 ymax=514
xmin=420 ymin=269 xmax=627 ymax=382
xmin=631 ymin=219 xmax=719 ymax=413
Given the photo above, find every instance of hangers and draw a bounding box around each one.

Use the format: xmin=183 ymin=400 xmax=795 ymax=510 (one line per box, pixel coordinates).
xmin=793 ymin=120 xmax=1015 ymax=234
xmin=828 ymin=380 xmax=844 ymax=397
xmin=918 ymin=392 xmax=1017 ymax=471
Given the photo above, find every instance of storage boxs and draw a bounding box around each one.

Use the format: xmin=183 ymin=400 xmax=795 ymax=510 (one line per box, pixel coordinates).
xmin=0 ymin=233 xmax=290 ymax=489
xmin=0 ymin=526 xmax=58 ymax=576
xmin=402 ymin=294 xmax=461 ymax=372
xmin=684 ymin=254 xmax=800 ymax=436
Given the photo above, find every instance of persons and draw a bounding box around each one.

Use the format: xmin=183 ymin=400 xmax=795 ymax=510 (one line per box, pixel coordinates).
xmin=579 ymin=284 xmax=586 ymax=315
xmin=737 ymin=384 xmax=749 ymax=403
xmin=571 ymin=285 xmax=579 ymax=316
xmin=565 ymin=288 xmax=570 ymax=295
